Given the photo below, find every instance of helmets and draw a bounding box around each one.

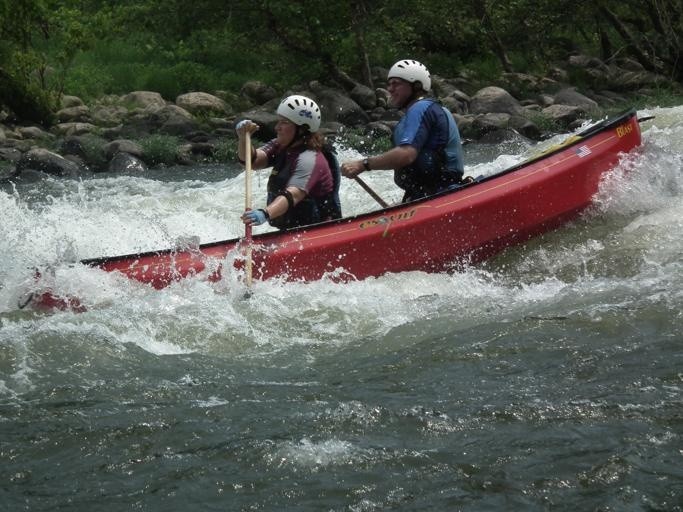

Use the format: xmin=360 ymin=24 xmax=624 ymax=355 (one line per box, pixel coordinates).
xmin=276 ymin=95 xmax=322 ymax=133
xmin=388 ymin=59 xmax=432 ymax=91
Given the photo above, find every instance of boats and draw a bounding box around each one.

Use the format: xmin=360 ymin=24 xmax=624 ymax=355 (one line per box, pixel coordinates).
xmin=29 ymin=108 xmax=642 ymax=315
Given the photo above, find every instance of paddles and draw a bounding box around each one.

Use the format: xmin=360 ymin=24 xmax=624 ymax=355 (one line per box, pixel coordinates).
xmin=244 ymin=133 xmax=252 ymax=300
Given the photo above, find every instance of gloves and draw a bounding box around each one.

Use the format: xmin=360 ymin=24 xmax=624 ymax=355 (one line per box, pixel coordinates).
xmin=242 ymin=208 xmax=269 ymax=227
xmin=235 ymin=119 xmax=260 ymax=140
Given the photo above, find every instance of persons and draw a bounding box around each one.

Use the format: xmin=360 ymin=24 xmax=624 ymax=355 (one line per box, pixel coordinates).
xmin=235 ymin=96 xmax=343 ymax=234
xmin=342 ymin=60 xmax=466 ymax=203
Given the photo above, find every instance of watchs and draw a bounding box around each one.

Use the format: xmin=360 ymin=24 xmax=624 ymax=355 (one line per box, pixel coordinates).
xmin=361 ymin=159 xmax=371 ymax=173
